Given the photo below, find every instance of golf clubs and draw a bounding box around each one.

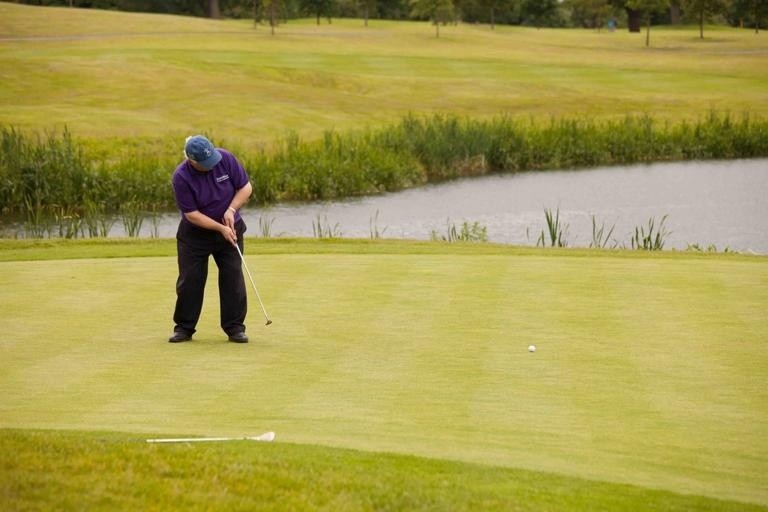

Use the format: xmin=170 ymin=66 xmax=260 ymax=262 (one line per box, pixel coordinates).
xmin=223 ymin=218 xmax=272 ymax=325
xmin=146 ymin=432 xmax=276 ymax=443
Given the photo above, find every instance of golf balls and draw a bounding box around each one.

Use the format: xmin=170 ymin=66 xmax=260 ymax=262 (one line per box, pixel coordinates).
xmin=529 ymin=345 xmax=536 ymax=352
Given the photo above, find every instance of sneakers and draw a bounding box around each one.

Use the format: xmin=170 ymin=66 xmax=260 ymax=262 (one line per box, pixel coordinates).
xmin=228 ymin=332 xmax=248 ymax=343
xmin=169 ymin=331 xmax=192 ymax=342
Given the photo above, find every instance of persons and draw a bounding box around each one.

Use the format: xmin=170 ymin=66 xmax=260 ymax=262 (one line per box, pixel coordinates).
xmin=168 ymin=134 xmax=253 ymax=344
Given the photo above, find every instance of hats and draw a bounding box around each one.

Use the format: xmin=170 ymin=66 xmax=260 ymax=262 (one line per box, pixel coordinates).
xmin=185 ymin=135 xmax=222 ymax=169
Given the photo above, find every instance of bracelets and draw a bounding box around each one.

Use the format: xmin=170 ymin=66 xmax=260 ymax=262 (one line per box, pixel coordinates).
xmin=227 ymin=206 xmax=236 ymax=216
